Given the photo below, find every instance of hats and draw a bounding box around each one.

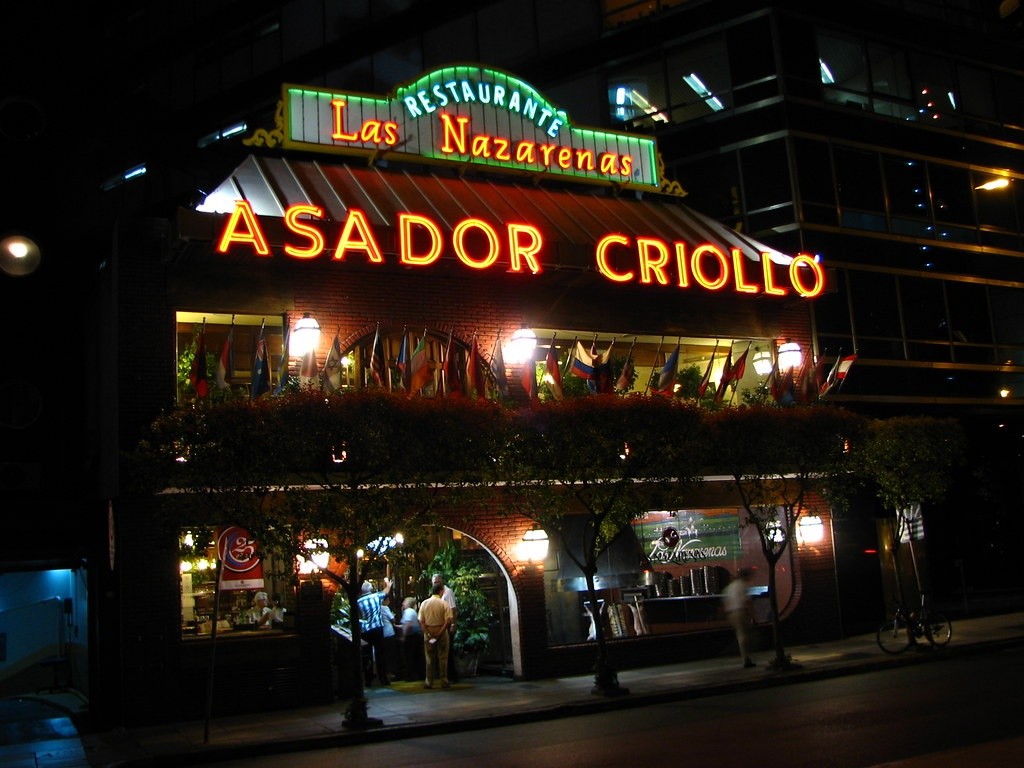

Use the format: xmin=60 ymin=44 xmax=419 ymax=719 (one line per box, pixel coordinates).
xmin=739 ymin=566 xmax=752 ymax=575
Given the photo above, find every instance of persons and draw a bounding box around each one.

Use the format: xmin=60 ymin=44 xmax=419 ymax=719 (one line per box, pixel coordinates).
xmin=380 ymin=595 xmax=395 ymax=637
xmin=245 ymin=592 xmax=272 ymax=626
xmin=357 ymin=578 xmax=394 ymax=688
xmin=724 ymin=566 xmax=757 ymax=667
xmin=395 ymin=597 xmax=425 ymax=682
xmin=432 ymin=574 xmax=461 ymax=684
xmin=418 ymin=584 xmax=454 ymax=687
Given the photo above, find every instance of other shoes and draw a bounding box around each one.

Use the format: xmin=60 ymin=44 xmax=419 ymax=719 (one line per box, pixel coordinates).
xmin=744 ymin=662 xmax=756 ymax=667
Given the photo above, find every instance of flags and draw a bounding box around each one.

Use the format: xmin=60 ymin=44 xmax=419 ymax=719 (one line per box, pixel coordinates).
xmin=217 ymin=332 xmax=233 ymax=389
xmin=466 ymin=337 xmax=483 ymax=392
xmin=444 ymin=336 xmax=459 ymax=394
xmin=490 ymin=340 xmax=507 ymax=400
xmin=191 ymin=333 xmax=209 ymax=396
xmin=370 ymin=327 xmax=385 ymax=388
xmin=250 ymin=336 xmax=270 ymax=398
xmin=396 ymin=331 xmax=410 ymax=391
xmin=273 ymin=324 xmax=289 ymax=395
xmin=542 ymin=340 xmax=858 ymax=405
xmin=408 ymin=337 xmax=428 ymax=399
xmin=520 ymin=362 xmax=538 ymax=399
xmin=323 ymin=331 xmax=342 ymax=398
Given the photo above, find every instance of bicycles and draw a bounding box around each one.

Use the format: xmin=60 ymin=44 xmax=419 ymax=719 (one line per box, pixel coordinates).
xmin=876 ymin=589 xmax=952 ymax=655
